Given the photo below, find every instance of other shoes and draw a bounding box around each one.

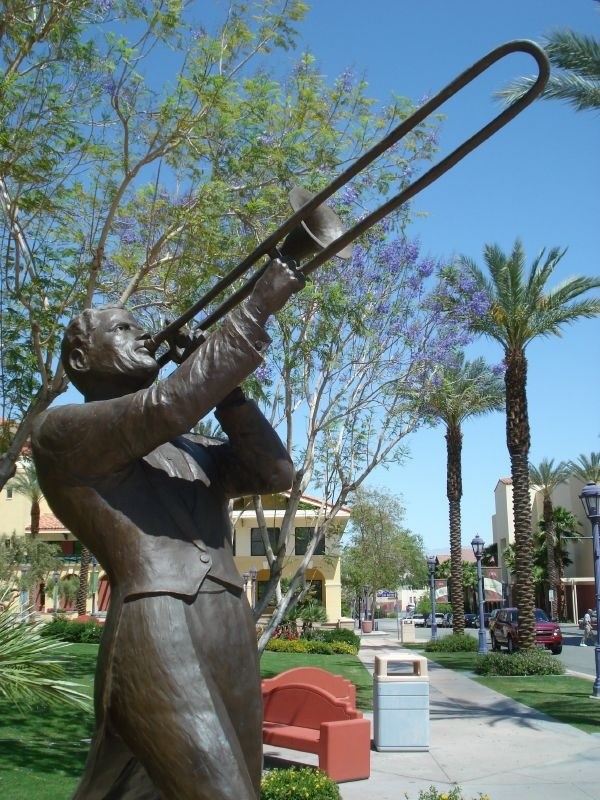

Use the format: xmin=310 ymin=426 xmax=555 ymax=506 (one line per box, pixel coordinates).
xmin=580 ymin=643 xmax=588 ymax=646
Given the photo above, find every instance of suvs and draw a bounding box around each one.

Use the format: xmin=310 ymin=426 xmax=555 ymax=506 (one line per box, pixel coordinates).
xmin=490 ymin=608 xmax=562 ymax=655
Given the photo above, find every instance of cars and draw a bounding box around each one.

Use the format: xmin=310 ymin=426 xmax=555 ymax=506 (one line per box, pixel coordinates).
xmin=401 ymin=613 xmax=488 ymax=627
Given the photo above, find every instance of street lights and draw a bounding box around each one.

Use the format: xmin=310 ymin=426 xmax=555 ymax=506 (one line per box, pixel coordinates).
xmin=53 ymin=571 xmax=60 ymax=614
xmin=579 ymin=477 xmax=600 ymax=698
xmin=357 ymin=582 xmax=369 ymax=629
xmin=242 ymin=566 xmax=259 ymax=611
xmin=471 ymin=532 xmax=490 ymax=655
xmin=426 ymin=555 xmax=439 ymax=642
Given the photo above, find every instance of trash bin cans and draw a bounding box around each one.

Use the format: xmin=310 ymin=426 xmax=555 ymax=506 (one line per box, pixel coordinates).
xmin=401 ymin=620 xmax=415 ymax=642
xmin=373 ymin=654 xmax=430 ymax=752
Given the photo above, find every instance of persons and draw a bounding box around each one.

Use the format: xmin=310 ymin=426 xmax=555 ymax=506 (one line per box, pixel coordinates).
xmin=31 ymin=258 xmax=306 ymax=799
xmin=580 ymin=609 xmax=593 ymax=646
